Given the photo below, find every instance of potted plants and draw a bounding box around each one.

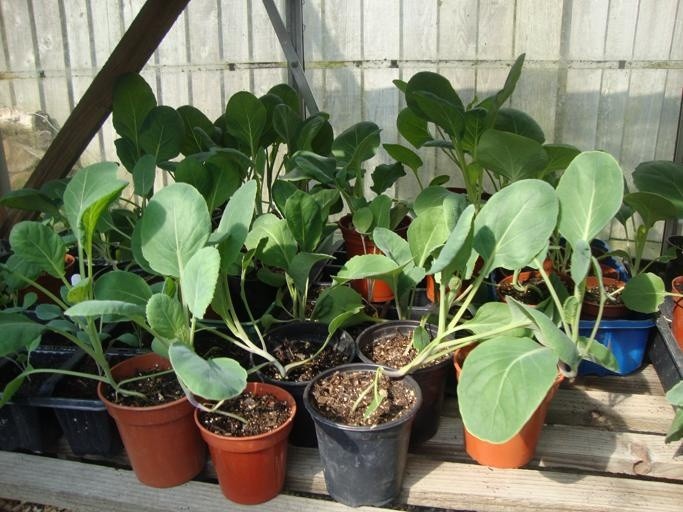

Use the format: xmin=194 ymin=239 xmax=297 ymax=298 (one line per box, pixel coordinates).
xmin=4 ymin=162 xmax=198 ymax=487
xmin=62 ymin=182 xmax=298 ymax=501
xmin=303 ymin=177 xmax=556 ymax=508
xmin=116 ymin=59 xmax=680 ymax=377
